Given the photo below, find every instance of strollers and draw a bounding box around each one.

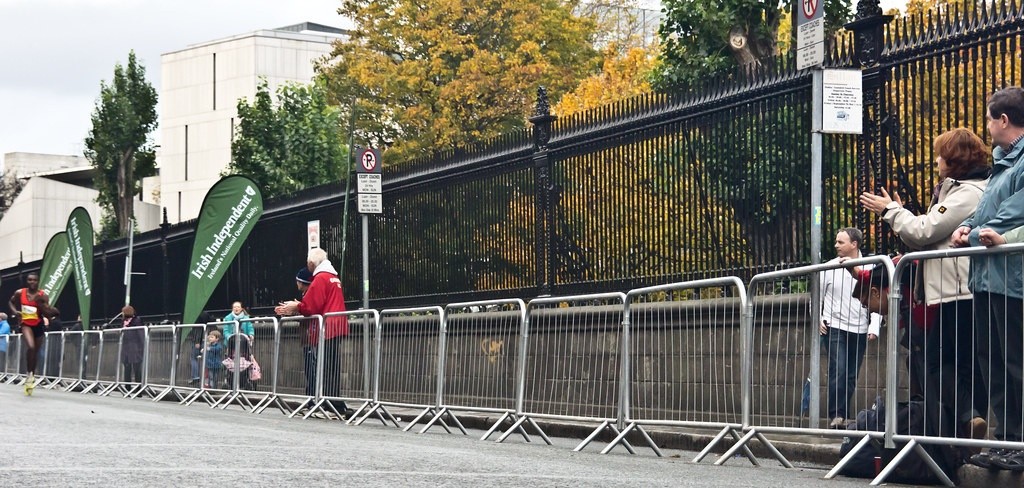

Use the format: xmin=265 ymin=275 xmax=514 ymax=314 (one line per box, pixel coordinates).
xmin=222 ymin=333 xmax=257 ymax=392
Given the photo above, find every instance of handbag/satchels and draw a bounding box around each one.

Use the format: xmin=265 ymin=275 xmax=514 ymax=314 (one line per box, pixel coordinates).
xmin=248 ymin=354 xmax=261 ymax=380
xmin=839 ymin=396 xmax=955 ymax=483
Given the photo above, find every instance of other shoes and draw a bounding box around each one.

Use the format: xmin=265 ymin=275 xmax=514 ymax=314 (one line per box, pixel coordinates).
xmin=970 ymin=448 xmax=998 ymax=468
xmin=829 ymin=416 xmax=844 ymax=429
xmin=315 ymin=408 xmax=346 ymax=420
xmin=300 ymin=406 xmax=320 ymax=417
xmin=965 ymin=417 xmax=987 ymax=454
xmin=137 ymin=393 xmax=142 ymax=397
xmin=990 ymin=450 xmax=1024 ymax=471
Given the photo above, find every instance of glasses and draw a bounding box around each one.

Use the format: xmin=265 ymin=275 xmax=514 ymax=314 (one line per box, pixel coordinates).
xmin=861 ymin=305 xmax=871 ymax=308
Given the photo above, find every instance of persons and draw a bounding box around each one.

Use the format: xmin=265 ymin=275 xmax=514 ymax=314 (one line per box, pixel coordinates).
xmin=222 ymin=302 xmax=255 ymax=348
xmin=274 ymin=267 xmax=317 ymax=407
xmin=69 ymin=315 xmax=97 ymax=391
xmin=121 ymin=306 xmax=144 ymax=397
xmin=8 ymin=271 xmax=60 ymax=396
xmin=282 ymin=247 xmax=348 ymax=416
xmin=188 ymin=310 xmax=216 ymax=385
xmin=809 ymin=226 xmax=884 ymax=429
xmin=837 ymin=85 xmax=1024 ymax=472
xmin=34 ymin=308 xmax=65 ymax=385
xmin=0 ymin=313 xmax=10 ymax=382
xmin=200 ymin=330 xmax=224 ymax=395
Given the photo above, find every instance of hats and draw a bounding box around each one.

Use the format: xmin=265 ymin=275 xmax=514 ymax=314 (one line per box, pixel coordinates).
xmin=296 ymin=267 xmax=313 ymax=283
xmin=852 ymin=270 xmax=871 ymax=304
